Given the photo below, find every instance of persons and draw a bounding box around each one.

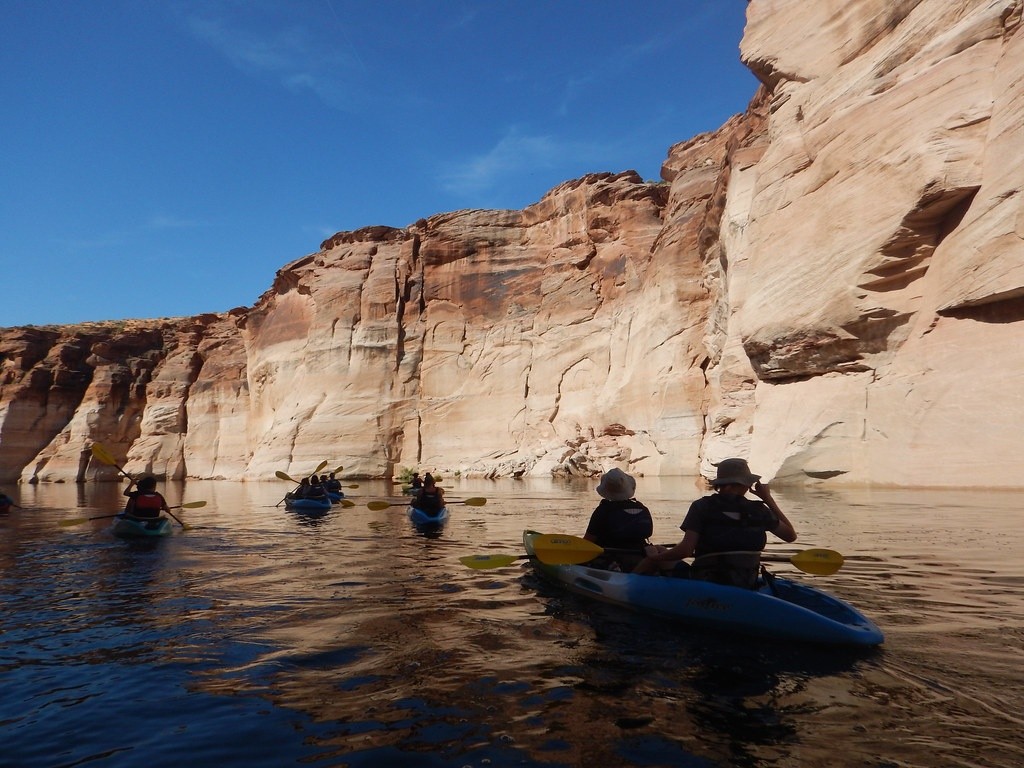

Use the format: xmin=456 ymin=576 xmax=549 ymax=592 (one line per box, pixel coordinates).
xmin=123 ymin=477 xmax=171 ymax=529
xmin=408 ymin=473 xmax=425 ymax=489
xmin=328 ymin=473 xmax=342 ymax=491
xmin=319 ymin=475 xmax=331 ymax=492
xmin=306 ymin=475 xmax=329 ymax=499
xmin=650 ymin=458 xmax=797 ymax=592
xmin=291 ymin=478 xmax=310 ymax=499
xmin=410 ymin=472 xmax=446 ymax=517
xmin=581 ymin=468 xmax=654 ymax=574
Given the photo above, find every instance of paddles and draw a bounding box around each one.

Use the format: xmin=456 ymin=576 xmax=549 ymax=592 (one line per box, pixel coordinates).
xmin=392 ymin=472 xmax=448 ymax=486
xmin=53 ymin=499 xmax=210 ymax=529
xmin=530 ymin=531 xmax=845 ymax=576
xmin=457 ymin=540 xmax=686 ymax=571
xmin=364 ymin=492 xmax=489 ymax=513
xmin=90 ymin=442 xmax=191 ymax=530
xmin=270 ymin=459 xmax=358 ymax=510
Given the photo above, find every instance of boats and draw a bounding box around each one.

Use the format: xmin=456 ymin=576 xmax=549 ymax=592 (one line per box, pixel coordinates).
xmin=0 ymin=494 xmax=13 ymax=515
xmin=110 ymin=511 xmax=174 ymax=541
xmin=405 ymin=504 xmax=451 ymax=527
xmin=521 ymin=526 xmax=887 ymax=647
xmin=329 ymin=492 xmax=346 ymax=503
xmin=284 ymin=491 xmax=332 ymax=512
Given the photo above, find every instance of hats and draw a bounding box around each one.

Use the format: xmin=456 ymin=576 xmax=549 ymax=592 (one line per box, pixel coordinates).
xmin=709 ymin=458 xmax=761 ymax=488
xmin=596 ymin=469 xmax=635 ymax=501
